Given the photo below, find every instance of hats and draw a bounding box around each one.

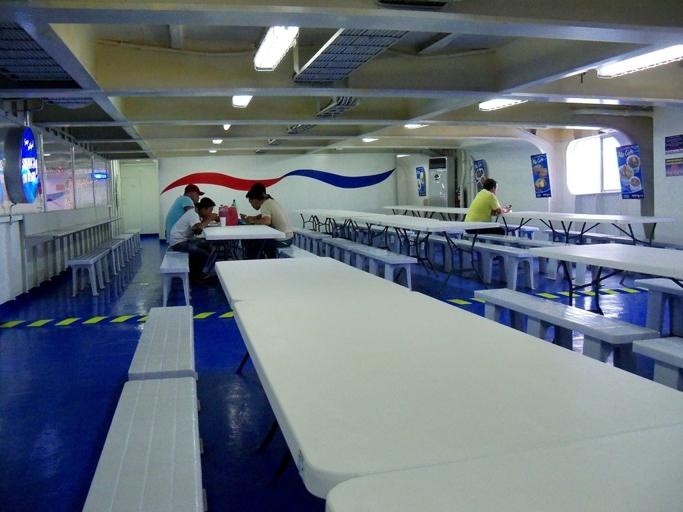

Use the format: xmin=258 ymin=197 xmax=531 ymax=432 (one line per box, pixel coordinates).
xmin=184 ymin=183 xmax=271 ymax=210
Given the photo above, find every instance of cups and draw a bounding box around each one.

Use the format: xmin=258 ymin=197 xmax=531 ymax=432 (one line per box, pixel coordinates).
xmin=220 ymin=216 xmax=226 ymax=227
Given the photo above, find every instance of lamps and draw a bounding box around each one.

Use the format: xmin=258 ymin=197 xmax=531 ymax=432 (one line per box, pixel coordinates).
xmin=597 ymin=44 xmax=683 ymax=79
xmin=360 ymin=123 xmax=428 ymax=159
xmin=477 ymin=98 xmax=527 ymax=111
xmin=254 ymin=26 xmax=301 ymax=72
xmin=207 ymin=94 xmax=252 ymax=155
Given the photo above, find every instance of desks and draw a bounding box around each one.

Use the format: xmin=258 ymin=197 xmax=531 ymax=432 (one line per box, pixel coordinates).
xmin=27 ymin=214 xmax=122 ymax=286
xmin=324 ymin=418 xmax=682 ymax=509
xmin=212 ymin=255 xmax=682 ymax=500
xmin=202 ymin=215 xmax=285 ymax=265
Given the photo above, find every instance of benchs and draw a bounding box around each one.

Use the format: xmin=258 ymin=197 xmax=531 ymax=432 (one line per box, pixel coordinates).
xmin=159 ymin=249 xmax=191 ymax=307
xmin=127 ymin=307 xmax=200 ymax=379
xmin=67 ymin=229 xmax=142 ymax=298
xmin=81 ymin=377 xmax=208 ymax=512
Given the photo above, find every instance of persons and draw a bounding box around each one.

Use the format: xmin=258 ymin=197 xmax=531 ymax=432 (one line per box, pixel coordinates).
xmin=240 ymin=188 xmax=294 ymax=258
xmin=464 ymin=178 xmax=512 ymax=260
xmin=164 ymin=184 xmax=208 ymax=246
xmin=252 ymin=183 xmax=288 ymax=224
xmin=168 ymin=198 xmax=217 ymax=280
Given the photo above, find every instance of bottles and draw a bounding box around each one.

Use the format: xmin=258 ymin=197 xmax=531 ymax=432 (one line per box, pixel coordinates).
xmin=231 ymin=199 xmax=237 ymax=207
